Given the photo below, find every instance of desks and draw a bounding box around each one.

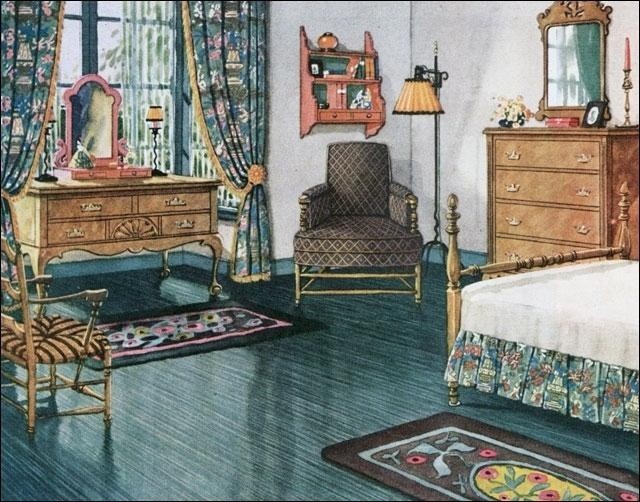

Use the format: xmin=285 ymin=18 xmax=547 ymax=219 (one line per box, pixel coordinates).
xmin=0 ymin=174 xmax=227 ymax=297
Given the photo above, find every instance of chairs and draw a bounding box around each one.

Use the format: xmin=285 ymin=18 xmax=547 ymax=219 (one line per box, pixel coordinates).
xmin=0 ymin=194 xmax=113 ymax=441
xmin=294 ymin=142 xmax=425 ymax=310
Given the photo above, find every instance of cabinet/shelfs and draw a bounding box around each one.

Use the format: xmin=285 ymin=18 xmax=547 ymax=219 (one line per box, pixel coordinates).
xmin=300 ymin=26 xmax=386 ymax=139
xmin=483 ymin=127 xmax=640 ymax=281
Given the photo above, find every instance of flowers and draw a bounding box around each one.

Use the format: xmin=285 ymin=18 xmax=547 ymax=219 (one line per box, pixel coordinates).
xmin=489 ymin=94 xmax=535 ymax=130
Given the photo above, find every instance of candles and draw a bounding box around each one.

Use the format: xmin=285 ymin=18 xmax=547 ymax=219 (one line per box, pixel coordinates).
xmin=624 ymin=37 xmax=631 ymax=70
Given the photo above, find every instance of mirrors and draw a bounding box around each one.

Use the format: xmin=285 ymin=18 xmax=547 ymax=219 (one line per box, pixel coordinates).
xmin=535 ymin=0 xmax=612 ymax=122
xmin=53 ymin=74 xmax=129 ymax=171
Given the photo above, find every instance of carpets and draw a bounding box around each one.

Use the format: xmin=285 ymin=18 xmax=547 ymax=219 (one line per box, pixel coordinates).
xmin=321 ymin=412 xmax=639 ymax=502
xmin=73 ymin=297 xmax=330 ymax=370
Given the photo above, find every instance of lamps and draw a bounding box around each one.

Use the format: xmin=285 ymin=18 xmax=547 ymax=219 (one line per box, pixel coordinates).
xmin=392 ymin=41 xmax=465 ymax=279
xmin=146 ymin=106 xmax=169 ymax=176
xmin=34 ymin=106 xmax=58 ymax=182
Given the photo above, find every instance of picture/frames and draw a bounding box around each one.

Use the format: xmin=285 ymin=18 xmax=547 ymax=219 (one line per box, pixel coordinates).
xmin=582 ymin=101 xmax=608 ymax=128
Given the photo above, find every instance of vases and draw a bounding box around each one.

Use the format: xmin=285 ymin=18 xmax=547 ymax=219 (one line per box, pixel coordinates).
xmin=498 ymin=119 xmax=524 ymax=127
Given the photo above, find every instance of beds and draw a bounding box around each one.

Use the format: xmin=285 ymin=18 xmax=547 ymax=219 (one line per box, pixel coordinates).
xmin=443 ymin=181 xmax=640 ymax=436
